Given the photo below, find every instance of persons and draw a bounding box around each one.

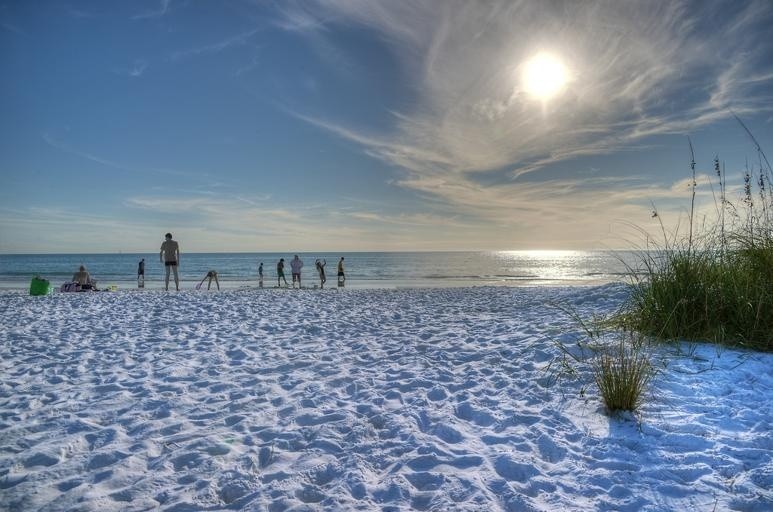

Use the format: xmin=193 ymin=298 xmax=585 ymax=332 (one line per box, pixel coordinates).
xmin=71 ymin=265 xmax=95 ymax=290
xmin=200 ymin=271 xmax=219 ymax=290
xmin=314 ymin=258 xmax=326 ymax=289
xmin=276 ymin=258 xmax=288 ymax=287
xmin=158 ymin=233 xmax=179 ymax=291
xmin=137 ymin=258 xmax=145 ymax=281
xmin=290 ymin=255 xmax=303 ymax=288
xmin=336 ymin=256 xmax=346 ymax=282
xmin=258 ymin=263 xmax=263 ymax=279
